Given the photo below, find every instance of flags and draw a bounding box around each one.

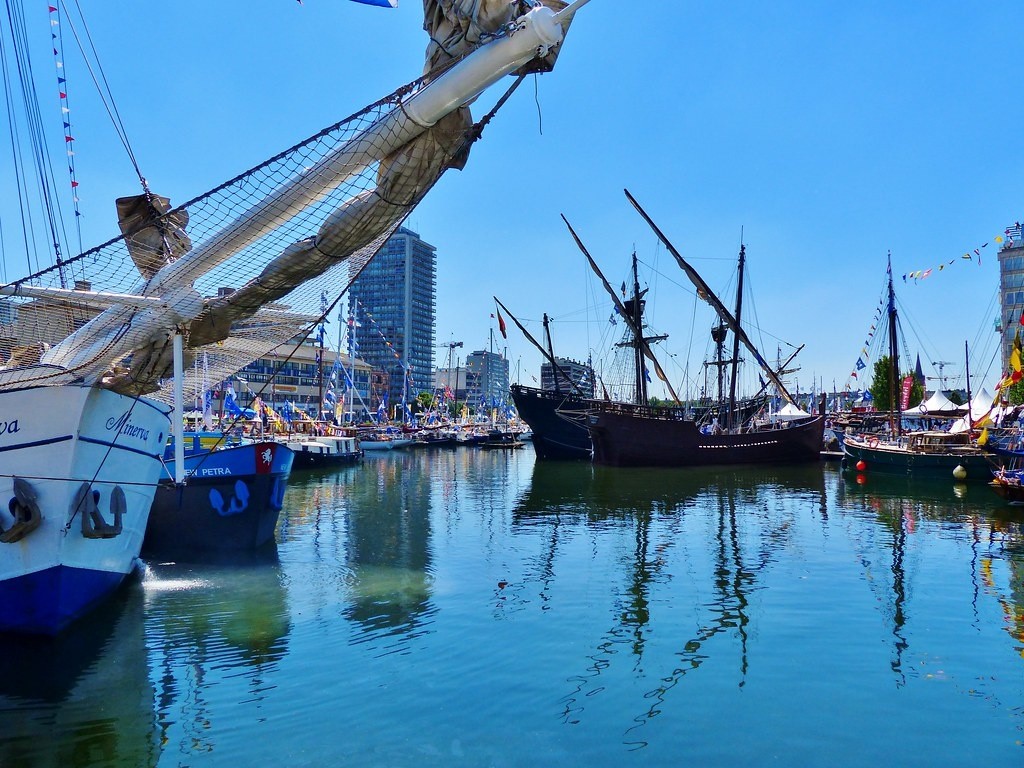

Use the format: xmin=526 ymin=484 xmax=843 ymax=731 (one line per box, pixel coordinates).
xmin=497 ymin=307 xmax=506 ymax=339
xmin=644 ymin=364 xmax=652 ymax=384
xmin=490 ymin=313 xmax=495 ymax=318
xmin=843 ymin=297 xmax=883 ymax=394
xmin=609 ymin=281 xmax=626 ymax=326
xmin=863 ymin=390 xmax=873 ymax=400
xmin=480 ymin=394 xmax=516 ymax=419
xmin=901 ymin=220 xmax=1020 ymax=286
xmin=971 ymin=306 xmax=1024 ymax=448
xmin=224 ymin=394 xmax=247 ymax=417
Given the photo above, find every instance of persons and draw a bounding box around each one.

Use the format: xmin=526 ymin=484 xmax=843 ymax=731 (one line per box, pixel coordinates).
xmin=776 ymin=420 xmax=796 ymax=429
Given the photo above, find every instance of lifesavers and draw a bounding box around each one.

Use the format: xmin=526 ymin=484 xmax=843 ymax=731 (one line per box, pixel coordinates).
xmin=870 ymin=437 xmax=880 ymax=450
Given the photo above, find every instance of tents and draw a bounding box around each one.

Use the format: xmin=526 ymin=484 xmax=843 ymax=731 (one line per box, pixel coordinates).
xmin=901 ymin=387 xmax=995 ymax=433
xmin=771 ymin=401 xmax=812 ymax=422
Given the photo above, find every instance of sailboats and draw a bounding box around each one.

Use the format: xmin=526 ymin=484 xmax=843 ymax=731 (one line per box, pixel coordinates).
xmin=1 ymin=0 xmax=1022 ymax=638
